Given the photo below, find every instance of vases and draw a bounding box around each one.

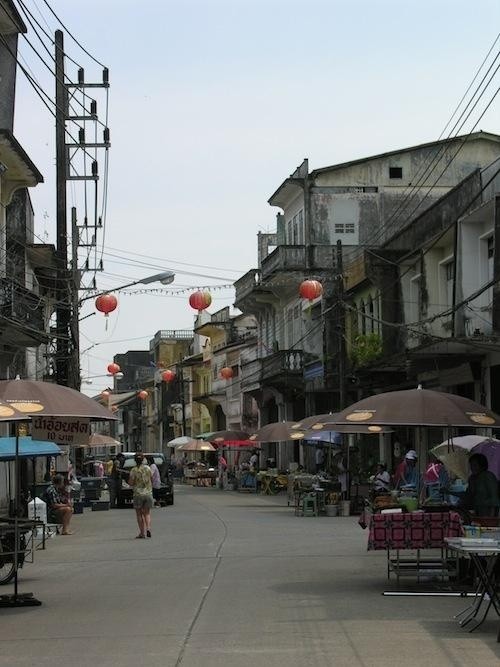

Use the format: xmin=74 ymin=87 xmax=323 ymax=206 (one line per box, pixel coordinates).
xmin=339 ymin=501 xmax=350 ymax=517
xmin=323 ymin=505 xmax=340 ymax=517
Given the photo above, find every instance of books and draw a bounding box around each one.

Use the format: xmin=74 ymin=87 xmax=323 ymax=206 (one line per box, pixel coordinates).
xmin=443 ymin=534 xmax=500 ymax=546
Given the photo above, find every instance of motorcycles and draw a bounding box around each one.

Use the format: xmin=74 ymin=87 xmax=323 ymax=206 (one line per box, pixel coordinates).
xmin=268 ymin=464 xmax=305 ymax=493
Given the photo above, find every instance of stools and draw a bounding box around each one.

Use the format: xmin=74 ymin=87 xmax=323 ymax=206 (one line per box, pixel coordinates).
xmin=301 ymin=498 xmax=317 ymax=517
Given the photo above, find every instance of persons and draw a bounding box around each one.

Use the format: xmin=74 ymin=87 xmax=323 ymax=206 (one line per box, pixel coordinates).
xmin=126 ymin=453 xmax=153 ymax=538
xmin=370 ymin=450 xmax=418 ymax=497
xmin=68 ymin=453 xmax=163 ymax=509
xmin=43 ymin=475 xmax=76 ymax=535
xmin=423 ymin=455 xmax=443 ymax=497
xmin=464 ymin=453 xmax=500 ymax=518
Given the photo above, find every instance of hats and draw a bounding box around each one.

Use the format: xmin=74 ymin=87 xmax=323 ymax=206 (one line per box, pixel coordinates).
xmin=406 ymin=450 xmax=418 ymax=459
xmin=134 ymin=453 xmax=144 ymax=460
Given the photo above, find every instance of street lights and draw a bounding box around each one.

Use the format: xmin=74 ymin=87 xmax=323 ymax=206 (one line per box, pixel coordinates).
xmin=72 ymin=271 xmax=177 ymax=392
xmin=81 ymin=380 xmax=93 ymax=385
xmin=80 ymin=372 xmax=125 ymax=380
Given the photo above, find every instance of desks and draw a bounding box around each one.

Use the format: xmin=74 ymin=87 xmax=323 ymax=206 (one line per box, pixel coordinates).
xmin=448 ymin=544 xmax=500 ymax=633
xmin=443 ymin=536 xmax=500 ymax=625
xmin=357 ymin=506 xmax=466 ymax=584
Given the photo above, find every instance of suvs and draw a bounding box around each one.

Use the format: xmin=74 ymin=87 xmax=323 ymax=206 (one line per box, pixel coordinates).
xmin=107 ymin=449 xmax=175 ymax=507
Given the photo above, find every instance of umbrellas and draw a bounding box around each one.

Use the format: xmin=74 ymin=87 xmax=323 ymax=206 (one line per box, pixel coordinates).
xmin=68 ymin=431 xmax=121 ymax=463
xmin=0 ymin=432 xmax=64 ymax=518
xmin=0 ymin=373 xmax=122 ymax=607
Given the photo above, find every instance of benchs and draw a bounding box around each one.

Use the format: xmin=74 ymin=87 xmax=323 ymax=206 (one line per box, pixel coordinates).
xmin=35 ymin=523 xmax=62 ymax=536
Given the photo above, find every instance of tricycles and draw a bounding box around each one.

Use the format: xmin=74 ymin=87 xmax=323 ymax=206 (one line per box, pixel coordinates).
xmin=1 ymin=517 xmax=46 ymax=587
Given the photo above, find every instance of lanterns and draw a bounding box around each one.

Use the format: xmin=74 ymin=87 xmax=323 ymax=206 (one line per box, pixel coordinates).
xmin=107 ymin=363 xmax=120 ymax=374
xmin=139 ymin=390 xmax=150 ymax=400
xmin=301 ymin=280 xmax=324 ymax=303
xmin=218 ymin=367 xmax=234 ymax=381
xmin=162 ymin=370 xmax=176 ymax=382
xmin=96 ymin=293 xmax=118 ymax=317
xmin=190 ymin=290 xmax=211 ymax=314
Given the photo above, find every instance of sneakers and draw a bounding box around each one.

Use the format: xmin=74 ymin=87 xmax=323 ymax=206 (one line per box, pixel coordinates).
xmin=146 ymin=529 xmax=151 ymax=537
